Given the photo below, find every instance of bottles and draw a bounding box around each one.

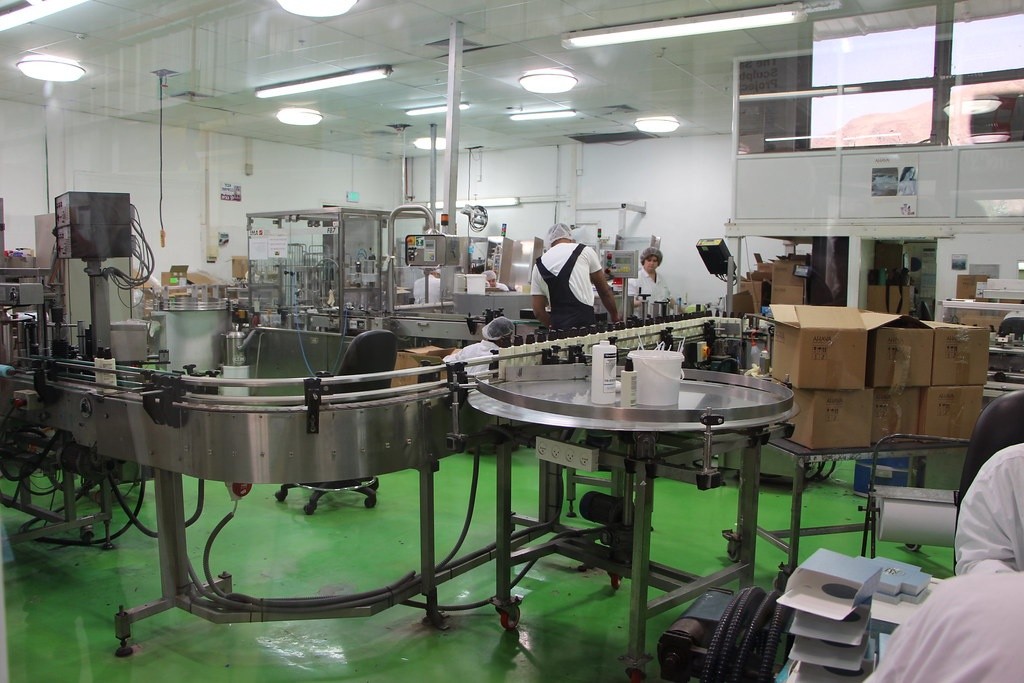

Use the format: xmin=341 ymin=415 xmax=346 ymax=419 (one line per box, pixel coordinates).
xmin=0 ymin=364 xmax=16 ymax=378
xmin=94 ymin=345 xmax=117 ymax=393
xmin=491 ymin=308 xmax=770 ymax=381
xmin=620 ymin=357 xmax=638 ymax=407
xmin=590 ymin=340 xmax=617 ymax=405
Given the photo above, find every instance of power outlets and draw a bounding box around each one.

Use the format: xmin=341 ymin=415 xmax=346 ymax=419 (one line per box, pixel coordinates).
xmin=536 ymin=437 xmax=599 ymax=473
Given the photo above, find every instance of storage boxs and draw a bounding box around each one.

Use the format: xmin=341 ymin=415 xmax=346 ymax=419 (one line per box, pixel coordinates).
xmin=957 ymin=274 xmax=991 ymax=301
xmin=774 ymin=545 xmax=882 ymax=682
xmin=227 ymin=256 xmax=248 ymax=278
xmin=730 ymin=251 xmax=812 ymax=315
xmin=748 ymin=306 xmax=868 ymax=388
xmin=161 ymin=263 xmax=214 ymax=293
xmin=392 ymin=345 xmax=465 ymax=389
xmin=861 ymin=310 xmax=935 ymax=388
xmin=920 ymin=385 xmax=985 ymax=444
xmin=923 ymin=319 xmax=991 ymax=386
xmin=872 ymin=387 xmax=923 ymax=444
xmin=785 ymin=386 xmax=871 ymax=449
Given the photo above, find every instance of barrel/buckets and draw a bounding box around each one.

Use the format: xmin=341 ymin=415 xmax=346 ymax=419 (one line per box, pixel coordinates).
xmin=627 ymin=349 xmax=685 ymax=406
xmin=853 ymin=457 xmax=910 ymax=493
xmin=220 ymin=330 xmax=247 ymax=366
xmin=453 ymin=274 xmax=466 ymax=292
xmin=222 ymin=366 xmax=249 ymax=397
xmin=466 ymin=274 xmax=486 ymax=295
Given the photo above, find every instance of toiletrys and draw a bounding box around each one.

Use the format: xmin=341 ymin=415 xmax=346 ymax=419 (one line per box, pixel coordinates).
xmin=488 ymin=334 xmax=526 ymax=381
xmin=548 ymin=326 xmax=589 ymax=358
xmin=620 ymin=356 xmax=639 ymax=408
xmin=590 ymin=340 xmax=617 ymax=404
xmin=588 ymin=314 xmax=644 ymax=355
xmin=524 ymin=330 xmax=549 ymax=365
xmin=713 ymin=307 xmax=749 ymax=338
xmin=659 ymin=326 xmax=674 ymax=351
xmin=541 ymin=343 xmax=588 ymax=365
xmin=608 ymin=335 xmax=619 ymax=365
xmin=644 ymin=303 xmax=712 ymax=345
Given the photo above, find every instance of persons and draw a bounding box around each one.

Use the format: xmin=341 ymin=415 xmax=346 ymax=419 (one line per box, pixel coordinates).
xmin=530 ymin=222 xmax=620 ymax=331
xmin=861 ymin=440 xmax=1024 ymax=683
xmin=481 ymin=270 xmax=510 ymax=291
xmin=627 ymin=246 xmax=676 ymax=318
xmin=413 ymin=268 xmax=441 ymax=304
xmin=443 ymin=316 xmax=515 ymax=383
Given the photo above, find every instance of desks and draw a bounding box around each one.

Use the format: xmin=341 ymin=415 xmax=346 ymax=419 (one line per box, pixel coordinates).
xmin=766 ymin=437 xmax=970 ymax=578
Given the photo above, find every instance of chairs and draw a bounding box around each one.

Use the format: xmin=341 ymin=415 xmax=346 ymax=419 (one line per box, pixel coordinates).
xmin=274 ymin=328 xmax=397 ymax=516
xmin=958 ymin=388 xmax=1023 ymax=514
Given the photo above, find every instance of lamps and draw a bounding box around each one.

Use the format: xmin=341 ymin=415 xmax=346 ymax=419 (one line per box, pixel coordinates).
xmin=254 ymin=0 xmax=809 ymax=150
xmin=16 ymin=54 xmax=88 ymax=83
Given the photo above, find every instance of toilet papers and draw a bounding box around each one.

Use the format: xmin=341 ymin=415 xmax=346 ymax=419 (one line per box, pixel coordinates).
xmin=875 ymin=497 xmax=958 ymax=548
